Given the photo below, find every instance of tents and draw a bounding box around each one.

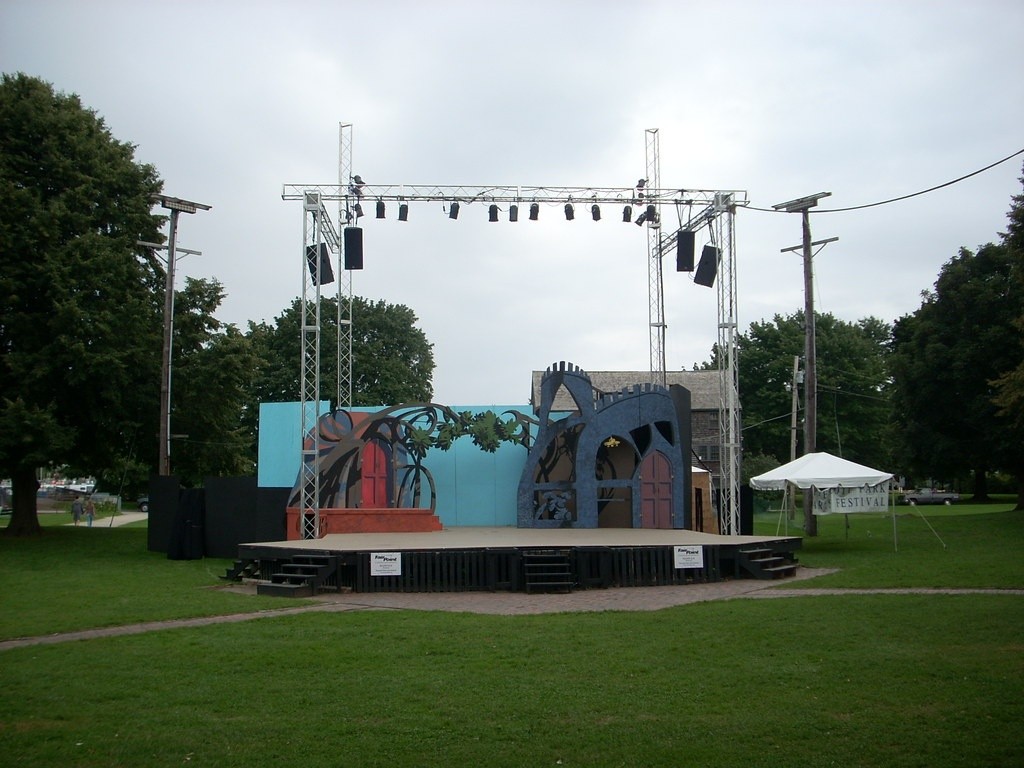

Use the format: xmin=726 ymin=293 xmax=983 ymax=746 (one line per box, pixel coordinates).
xmin=749 ymin=451 xmax=898 ymax=551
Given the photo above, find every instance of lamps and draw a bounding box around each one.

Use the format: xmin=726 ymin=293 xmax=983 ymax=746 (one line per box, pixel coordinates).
xmin=341 ymin=209 xmax=353 ymax=219
xmin=509 ymin=202 xmax=519 ymax=222
xmin=355 ymin=203 xmax=364 ymax=217
xmin=623 ymin=206 xmax=632 ymax=222
xmin=529 ymin=202 xmax=539 ymax=220
xmin=398 ymin=201 xmax=408 ymax=221
xmin=635 ymin=211 xmax=647 ymax=226
xmin=488 ymin=205 xmax=498 ymax=221
xmin=376 ymin=196 xmax=385 ymax=218
xmin=646 ymin=205 xmax=657 ymax=221
xmin=565 ymin=204 xmax=574 ymax=220
xmin=592 ymin=205 xmax=601 ymax=221
xmin=638 ymin=193 xmax=647 ymax=202
xmin=636 ymin=179 xmax=648 ymax=191
xmin=449 ymin=200 xmax=460 ymax=219
xmin=350 ymin=175 xmax=365 ymax=188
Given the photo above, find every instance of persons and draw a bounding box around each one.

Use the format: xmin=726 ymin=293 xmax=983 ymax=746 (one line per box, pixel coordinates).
xmin=71 ymin=495 xmax=84 ymax=526
xmin=84 ymin=500 xmax=96 ymax=526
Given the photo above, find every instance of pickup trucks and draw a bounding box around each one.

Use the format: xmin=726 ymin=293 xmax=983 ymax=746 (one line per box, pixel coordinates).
xmin=898 ymin=488 xmax=959 ymax=506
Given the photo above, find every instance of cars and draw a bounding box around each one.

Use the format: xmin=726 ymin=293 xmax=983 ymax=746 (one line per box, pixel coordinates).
xmin=136 ymin=497 xmax=148 ymax=512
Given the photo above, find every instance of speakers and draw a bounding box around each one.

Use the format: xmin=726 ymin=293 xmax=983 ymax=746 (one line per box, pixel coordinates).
xmin=694 ymin=245 xmax=723 ymax=289
xmin=344 ymin=227 xmax=363 ymax=270
xmin=677 ymin=230 xmax=696 ymax=272
xmin=307 ymin=243 xmax=333 ymax=287
